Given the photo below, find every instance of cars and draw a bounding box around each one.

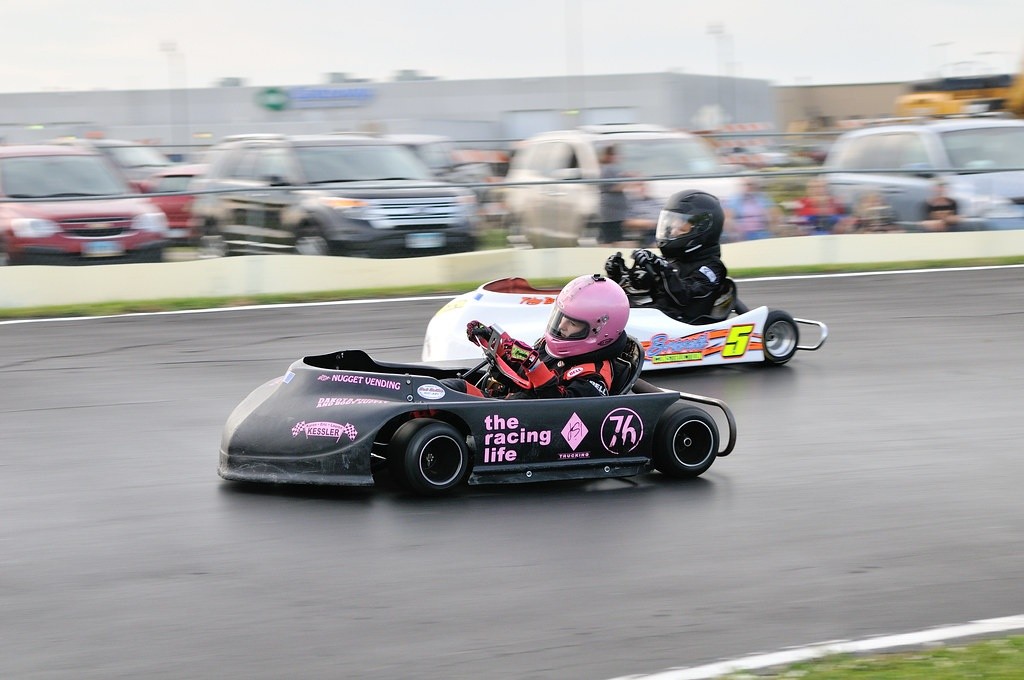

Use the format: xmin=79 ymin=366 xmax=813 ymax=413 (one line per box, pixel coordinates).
xmin=53 ymin=137 xmax=519 ymax=230
xmin=0 ymin=147 xmax=172 ymax=265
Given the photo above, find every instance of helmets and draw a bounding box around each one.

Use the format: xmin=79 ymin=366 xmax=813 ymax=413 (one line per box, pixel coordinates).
xmin=545 ymin=274 xmax=630 ymax=359
xmin=655 ymin=189 xmax=725 ymax=259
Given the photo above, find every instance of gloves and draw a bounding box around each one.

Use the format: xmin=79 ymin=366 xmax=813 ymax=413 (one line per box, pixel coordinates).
xmin=604 ymin=252 xmax=629 ymax=278
xmin=502 ymin=338 xmax=540 ymax=370
xmin=632 ymin=249 xmax=670 ymax=273
xmin=466 ymin=320 xmax=497 ymax=350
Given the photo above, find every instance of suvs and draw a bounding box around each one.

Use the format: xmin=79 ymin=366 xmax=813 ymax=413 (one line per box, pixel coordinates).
xmin=507 ymin=124 xmax=770 ymax=249
xmin=812 ymin=115 xmax=1024 ymax=228
xmin=187 ymin=134 xmax=484 ymax=259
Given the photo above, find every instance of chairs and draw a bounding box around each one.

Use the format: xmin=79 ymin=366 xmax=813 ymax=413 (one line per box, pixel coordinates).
xmin=609 ymin=336 xmax=645 ymax=398
xmin=690 ymin=278 xmax=737 ymax=325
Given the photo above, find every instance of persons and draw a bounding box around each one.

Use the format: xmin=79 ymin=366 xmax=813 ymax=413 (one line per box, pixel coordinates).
xmin=593 ymin=171 xmax=966 ymax=248
xmin=605 ymin=189 xmax=727 ymax=327
xmin=439 ymin=274 xmax=631 ymax=401
